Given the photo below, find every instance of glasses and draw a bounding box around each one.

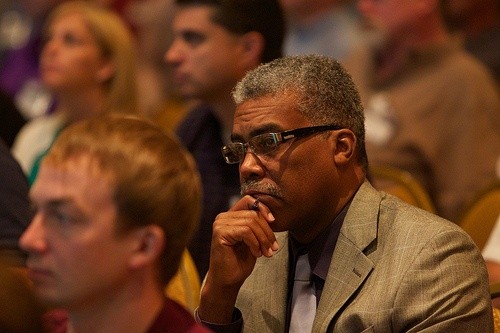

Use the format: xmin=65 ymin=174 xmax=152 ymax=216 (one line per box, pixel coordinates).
xmin=220 ymin=125 xmax=342 ymax=165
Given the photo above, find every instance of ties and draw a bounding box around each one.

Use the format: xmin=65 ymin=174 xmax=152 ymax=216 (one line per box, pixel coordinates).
xmin=288 ymin=254 xmax=317 ymax=333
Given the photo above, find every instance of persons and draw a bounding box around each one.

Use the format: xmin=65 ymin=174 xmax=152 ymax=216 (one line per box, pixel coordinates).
xmin=194 ymin=54 xmax=493 ymax=333
xmin=162 ymin=0 xmax=280 ymax=203
xmin=15 ymin=114 xmax=207 ymax=333
xmin=13 ymin=0 xmax=149 ymax=183
xmin=326 ymin=1 xmax=497 ymax=246
